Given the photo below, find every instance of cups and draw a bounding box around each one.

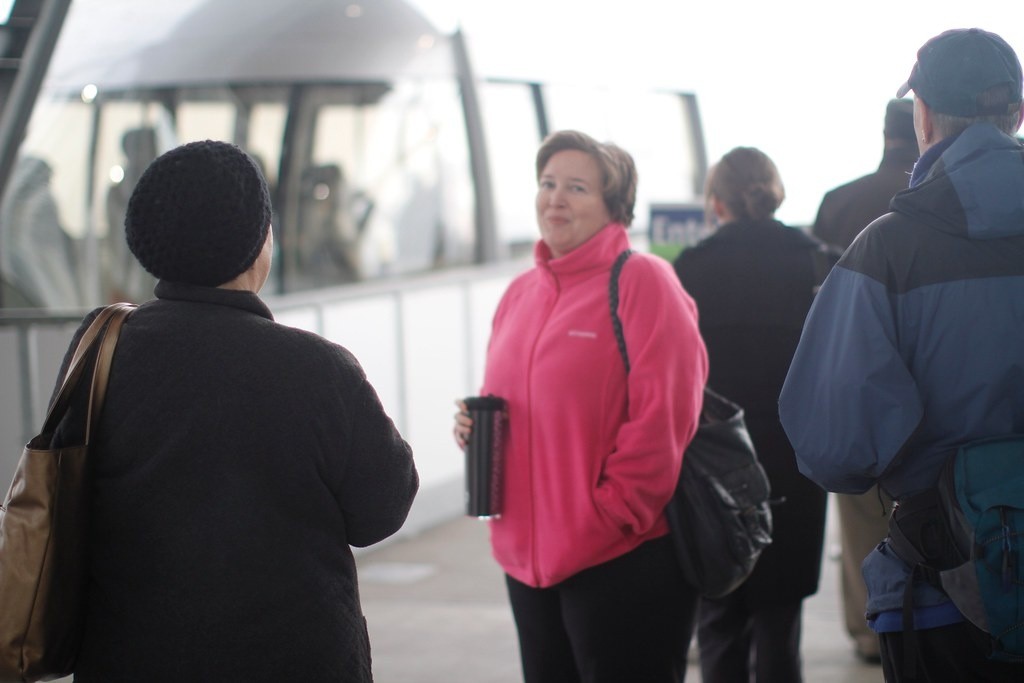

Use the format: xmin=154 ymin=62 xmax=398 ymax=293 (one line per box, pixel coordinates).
xmin=462 ymin=397 xmax=508 ymax=518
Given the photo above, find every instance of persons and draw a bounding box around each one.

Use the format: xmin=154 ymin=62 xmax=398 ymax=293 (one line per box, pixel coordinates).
xmin=22 ymin=138 xmax=421 ymax=683
xmin=668 ymin=145 xmax=845 ymax=683
xmin=810 ymin=97 xmax=922 ymax=665
xmin=100 ymin=120 xmax=169 ymax=301
xmin=777 ymin=27 xmax=1024 ymax=682
xmin=450 ymin=129 xmax=710 ymax=683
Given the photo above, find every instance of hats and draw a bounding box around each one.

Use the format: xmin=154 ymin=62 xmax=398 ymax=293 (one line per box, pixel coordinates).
xmin=896 ymin=28 xmax=1023 ymax=117
xmin=884 ymin=98 xmax=916 ymax=140
xmin=125 ymin=140 xmax=272 ymax=286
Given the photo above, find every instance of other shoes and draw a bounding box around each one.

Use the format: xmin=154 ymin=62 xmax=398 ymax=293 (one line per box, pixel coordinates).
xmin=855 ymin=643 xmax=882 ymax=666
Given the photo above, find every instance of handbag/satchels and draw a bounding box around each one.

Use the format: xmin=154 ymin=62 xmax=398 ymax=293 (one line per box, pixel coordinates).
xmin=608 ymin=247 xmax=773 ymax=601
xmin=886 ymin=434 xmax=1024 ymax=683
xmin=0 ymin=302 xmax=137 ymax=683
